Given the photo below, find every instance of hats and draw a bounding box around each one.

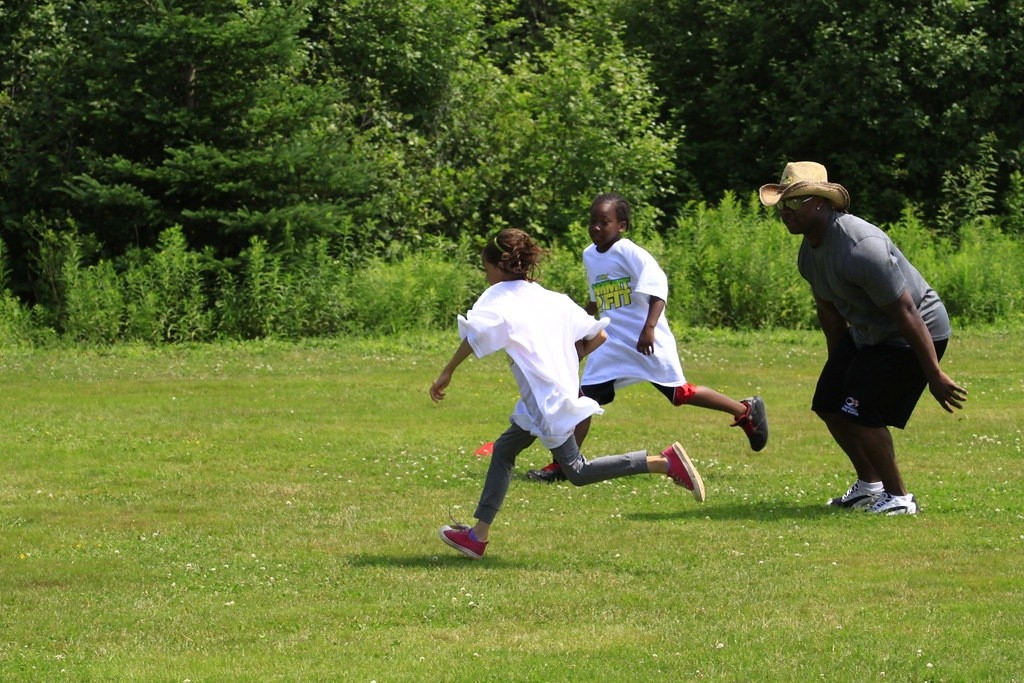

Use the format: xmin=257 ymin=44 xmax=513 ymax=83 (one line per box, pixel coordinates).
xmin=759 ymin=161 xmax=850 ymax=213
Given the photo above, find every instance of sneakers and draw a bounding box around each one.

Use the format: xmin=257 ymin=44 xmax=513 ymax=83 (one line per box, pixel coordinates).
xmin=439 ymin=525 xmax=489 ymax=560
xmin=826 ymin=478 xmax=885 ymax=508
xmin=658 ymin=441 xmax=705 ymax=502
xmin=729 ymin=396 xmax=769 ymax=452
xmin=526 ymin=462 xmax=567 ymax=481
xmin=863 ymin=494 xmax=919 ymax=516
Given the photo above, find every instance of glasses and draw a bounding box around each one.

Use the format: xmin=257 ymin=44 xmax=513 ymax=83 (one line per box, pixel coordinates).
xmin=776 ymin=196 xmax=815 ymax=209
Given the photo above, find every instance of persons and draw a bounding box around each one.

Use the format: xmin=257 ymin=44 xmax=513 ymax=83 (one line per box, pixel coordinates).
xmin=525 ymin=193 xmax=768 ymax=482
xmin=759 ymin=161 xmax=969 ymax=515
xmin=430 ymin=231 xmax=704 ymax=562
